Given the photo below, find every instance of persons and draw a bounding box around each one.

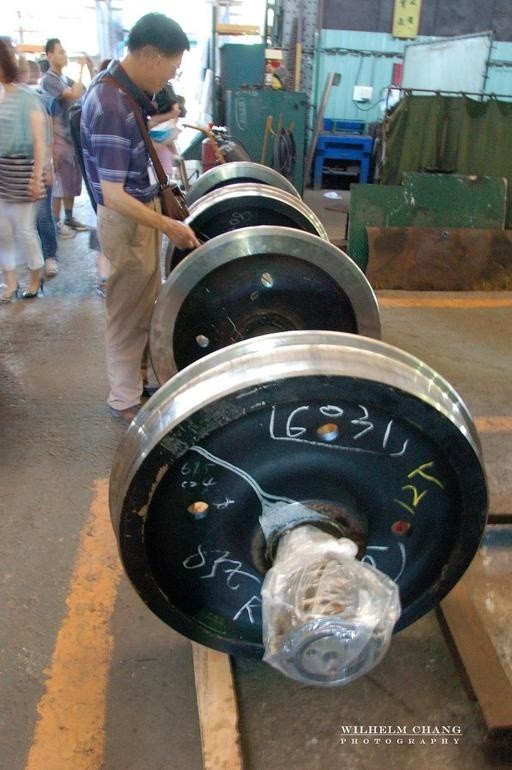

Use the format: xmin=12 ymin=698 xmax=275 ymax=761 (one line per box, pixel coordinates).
xmin=146 ymin=84 xmax=180 ymax=155
xmin=78 ymin=12 xmax=202 ymax=426
xmin=1 ymin=36 xmax=111 ymax=305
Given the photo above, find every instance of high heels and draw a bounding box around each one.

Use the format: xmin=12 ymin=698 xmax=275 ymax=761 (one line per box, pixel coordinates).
xmin=0 ymin=257 xmax=57 ymax=305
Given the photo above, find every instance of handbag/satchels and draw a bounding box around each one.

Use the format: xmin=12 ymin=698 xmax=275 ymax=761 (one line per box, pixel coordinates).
xmin=161 ymin=182 xmax=190 ymax=223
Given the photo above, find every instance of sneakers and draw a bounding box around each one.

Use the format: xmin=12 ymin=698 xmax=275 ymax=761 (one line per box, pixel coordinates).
xmin=56 ymin=218 xmax=89 ymax=239
xmin=110 ymin=405 xmax=141 ymax=425
